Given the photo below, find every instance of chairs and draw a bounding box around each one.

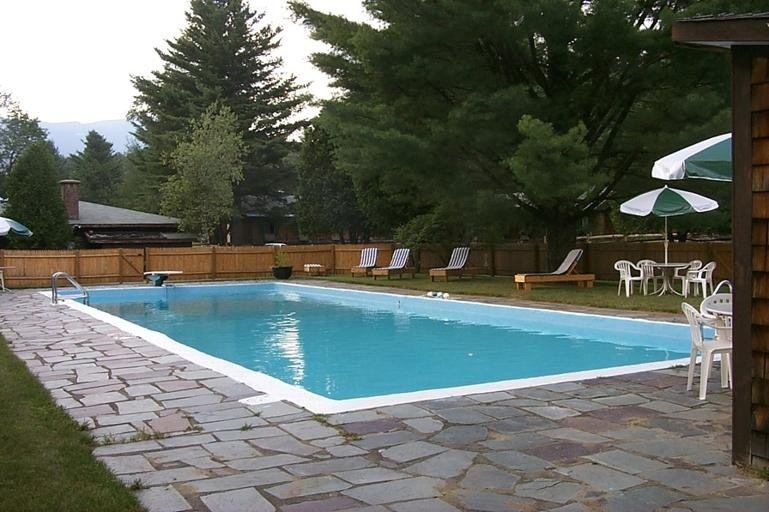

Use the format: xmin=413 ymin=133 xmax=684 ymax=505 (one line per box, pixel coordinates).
xmin=373 ymin=248 xmax=416 ymax=281
xmin=304 ymin=263 xmax=326 ymax=276
xmin=515 ymin=249 xmax=596 ymax=291
xmin=615 ymin=258 xmax=733 ymax=400
xmin=351 ymin=248 xmax=385 ymax=278
xmin=429 ymin=247 xmax=479 ymax=282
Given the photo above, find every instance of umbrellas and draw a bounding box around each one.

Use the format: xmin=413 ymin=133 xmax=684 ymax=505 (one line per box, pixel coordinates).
xmin=620 ymin=184 xmax=721 ymax=262
xmin=0 ymin=217 xmax=33 ymax=238
xmin=651 ymin=133 xmax=733 ymax=183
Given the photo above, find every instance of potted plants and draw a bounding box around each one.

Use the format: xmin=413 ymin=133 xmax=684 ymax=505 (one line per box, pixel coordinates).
xmin=271 ymin=250 xmax=292 ymax=279
